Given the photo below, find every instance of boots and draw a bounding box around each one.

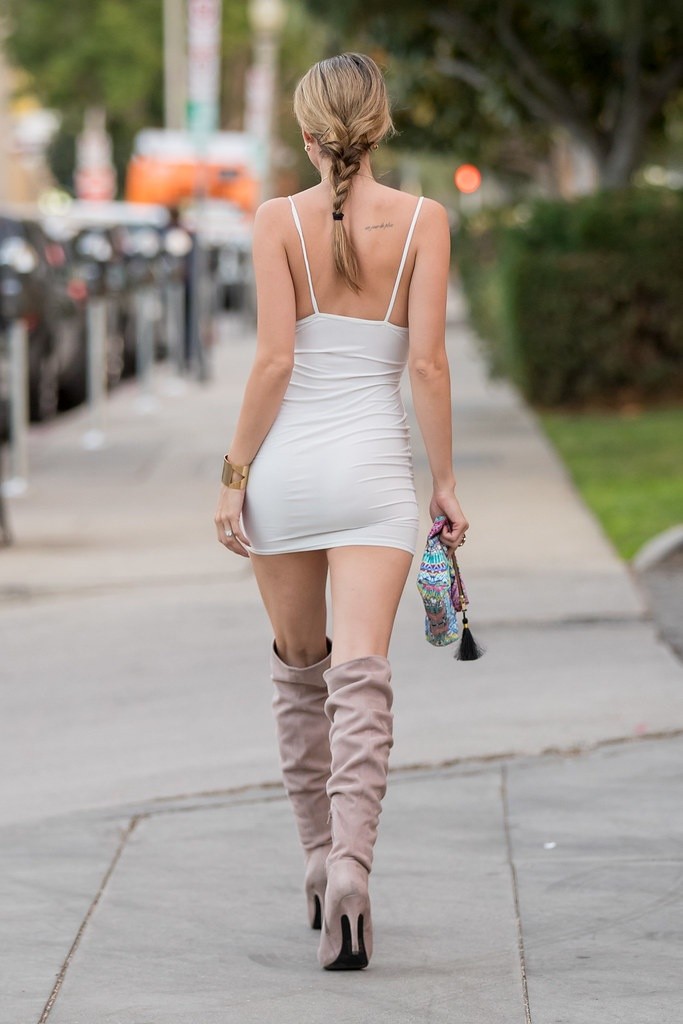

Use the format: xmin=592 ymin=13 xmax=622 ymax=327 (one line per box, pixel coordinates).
xmin=317 ymin=657 xmax=393 ymax=970
xmin=273 ymin=636 xmax=332 ymax=929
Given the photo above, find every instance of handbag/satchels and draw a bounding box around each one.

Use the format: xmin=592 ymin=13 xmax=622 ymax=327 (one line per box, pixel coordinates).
xmin=417 ymin=516 xmax=483 ymax=660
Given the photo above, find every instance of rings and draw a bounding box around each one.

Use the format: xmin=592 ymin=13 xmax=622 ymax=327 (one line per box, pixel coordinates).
xmin=224 ymin=530 xmax=233 ymax=537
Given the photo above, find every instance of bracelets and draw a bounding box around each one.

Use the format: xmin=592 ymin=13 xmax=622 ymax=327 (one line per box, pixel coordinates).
xmin=221 ymin=454 xmax=250 ymax=490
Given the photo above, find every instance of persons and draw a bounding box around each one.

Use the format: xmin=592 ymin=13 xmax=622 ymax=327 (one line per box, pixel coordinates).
xmin=215 ymin=51 xmax=470 ymax=970
